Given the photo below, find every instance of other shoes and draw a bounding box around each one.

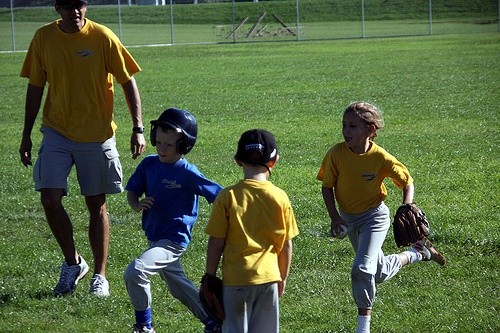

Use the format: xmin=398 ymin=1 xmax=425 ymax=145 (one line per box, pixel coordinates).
xmin=411 ymin=232 xmax=448 ymax=265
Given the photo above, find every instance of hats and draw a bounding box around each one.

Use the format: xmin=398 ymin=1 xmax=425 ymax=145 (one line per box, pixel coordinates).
xmin=236 ymin=125 xmax=278 ymax=166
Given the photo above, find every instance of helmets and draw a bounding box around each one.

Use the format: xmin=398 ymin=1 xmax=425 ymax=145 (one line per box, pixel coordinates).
xmin=149 ymin=105 xmax=199 ymax=158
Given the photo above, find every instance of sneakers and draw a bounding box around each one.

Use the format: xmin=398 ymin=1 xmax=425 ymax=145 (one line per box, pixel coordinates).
xmin=88 ymin=273 xmax=110 ymax=301
xmin=54 ymin=252 xmax=89 ymax=299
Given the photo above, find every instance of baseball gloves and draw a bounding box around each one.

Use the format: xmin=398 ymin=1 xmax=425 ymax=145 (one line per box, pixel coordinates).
xmin=198 ymin=273 xmax=225 ymax=323
xmin=392 ymin=203 xmax=430 ymax=249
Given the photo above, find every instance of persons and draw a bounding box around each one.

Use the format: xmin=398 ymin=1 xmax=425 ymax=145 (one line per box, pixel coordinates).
xmin=124 ymin=108 xmax=225 ymax=333
xmin=317 ymin=102 xmax=446 ymax=333
xmin=20 ymin=0 xmax=146 ymax=296
xmin=200 ymin=129 xmax=299 ymax=333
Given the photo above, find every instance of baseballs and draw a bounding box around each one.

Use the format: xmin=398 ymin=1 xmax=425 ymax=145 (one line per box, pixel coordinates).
xmin=333 ymin=225 xmax=348 ymax=239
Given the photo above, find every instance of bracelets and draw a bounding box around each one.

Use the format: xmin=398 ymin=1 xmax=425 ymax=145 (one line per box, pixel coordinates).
xmin=133 ymin=127 xmax=145 ymax=133
